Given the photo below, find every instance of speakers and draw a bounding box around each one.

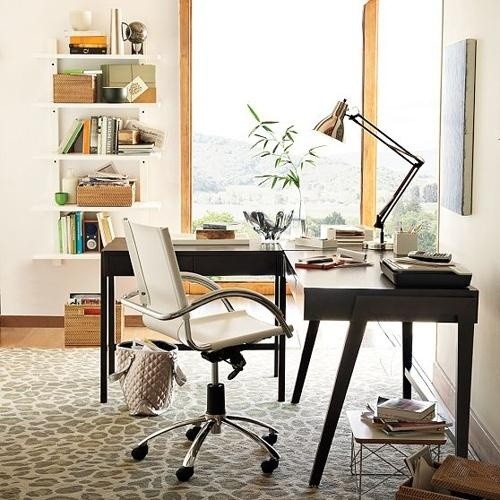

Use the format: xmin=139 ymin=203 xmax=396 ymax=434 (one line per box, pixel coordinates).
xmin=83 ymin=220 xmax=101 ymax=252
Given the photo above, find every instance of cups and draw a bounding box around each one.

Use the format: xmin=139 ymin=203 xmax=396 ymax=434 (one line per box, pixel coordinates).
xmin=69 ymin=10 xmax=92 ymax=31
xmin=55 ymin=192 xmax=69 ymax=206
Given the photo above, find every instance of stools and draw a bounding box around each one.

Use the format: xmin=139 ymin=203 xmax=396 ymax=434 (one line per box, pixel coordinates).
xmin=348 ymin=408 xmax=447 ymax=500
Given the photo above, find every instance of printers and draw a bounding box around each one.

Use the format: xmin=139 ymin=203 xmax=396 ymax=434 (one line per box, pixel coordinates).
xmin=380 ymin=258 xmax=473 ymax=288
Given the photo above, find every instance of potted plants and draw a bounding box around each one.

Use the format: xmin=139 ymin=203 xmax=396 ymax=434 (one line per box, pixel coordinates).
xmin=244 ymin=103 xmax=327 ymax=238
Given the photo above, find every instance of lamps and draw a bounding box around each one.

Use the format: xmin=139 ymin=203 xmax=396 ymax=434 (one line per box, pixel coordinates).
xmin=314 ymin=96 xmax=424 ymax=251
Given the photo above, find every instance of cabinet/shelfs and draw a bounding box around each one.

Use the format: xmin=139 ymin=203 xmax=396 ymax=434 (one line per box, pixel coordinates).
xmin=30 ymin=54 xmax=164 ymax=259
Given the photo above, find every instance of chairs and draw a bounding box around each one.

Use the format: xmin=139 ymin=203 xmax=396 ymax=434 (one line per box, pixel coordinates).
xmin=121 ymin=215 xmax=294 ymax=484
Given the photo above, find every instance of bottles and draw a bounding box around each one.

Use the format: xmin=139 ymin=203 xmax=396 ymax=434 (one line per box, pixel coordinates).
xmin=62 ymin=168 xmax=76 ymax=204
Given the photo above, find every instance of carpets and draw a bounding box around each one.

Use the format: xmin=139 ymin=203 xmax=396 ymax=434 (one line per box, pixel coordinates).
xmin=0 ymin=344 xmax=474 ymax=500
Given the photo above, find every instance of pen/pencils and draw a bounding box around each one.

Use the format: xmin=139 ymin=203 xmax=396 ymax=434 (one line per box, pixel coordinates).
xmin=399 ymin=222 xmax=402 ymax=232
xmin=408 ymin=224 xmax=422 ymax=234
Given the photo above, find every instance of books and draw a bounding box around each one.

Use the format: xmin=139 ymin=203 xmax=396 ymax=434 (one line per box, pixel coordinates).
xmin=68 ymin=30 xmax=110 ymax=55
xmin=292 ymin=225 xmax=372 ymax=270
xmin=196 ymin=231 xmax=235 ymax=240
xmin=67 ymin=292 xmax=103 ymax=316
xmin=56 ymin=116 xmax=167 ymax=254
xmin=403 ymin=445 xmax=432 ymax=478
xmin=202 ymin=223 xmax=227 ymax=230
xmin=360 ymin=396 xmax=453 ymax=437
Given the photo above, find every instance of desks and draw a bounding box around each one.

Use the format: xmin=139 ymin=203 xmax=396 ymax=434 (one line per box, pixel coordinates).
xmin=101 ymin=238 xmax=286 ymax=403
xmin=284 ymin=234 xmax=479 ymax=486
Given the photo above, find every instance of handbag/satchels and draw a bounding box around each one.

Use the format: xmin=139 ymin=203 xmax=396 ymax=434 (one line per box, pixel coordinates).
xmin=109 ymin=340 xmax=186 ymax=416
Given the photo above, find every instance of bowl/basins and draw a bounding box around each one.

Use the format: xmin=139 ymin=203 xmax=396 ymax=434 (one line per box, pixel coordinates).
xmin=102 ymin=86 xmax=127 ymax=104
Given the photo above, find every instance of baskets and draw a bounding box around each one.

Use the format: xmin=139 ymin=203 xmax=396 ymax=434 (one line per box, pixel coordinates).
xmin=76 ymin=181 xmax=135 ymax=207
xmin=64 ymin=302 xmax=124 ymax=346
xmin=54 ymin=73 xmax=96 ymax=102
